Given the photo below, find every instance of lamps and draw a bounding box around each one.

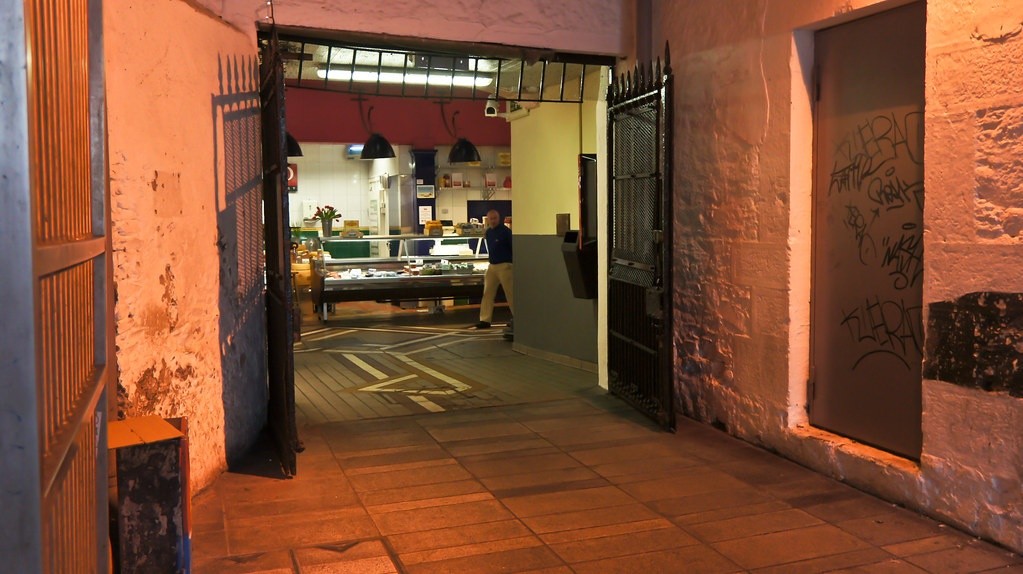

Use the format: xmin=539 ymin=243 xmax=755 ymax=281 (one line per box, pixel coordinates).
xmin=350 ymin=94 xmax=397 ymax=159
xmin=432 ymin=98 xmax=482 ymax=162
xmin=286 ymin=131 xmax=303 ymax=156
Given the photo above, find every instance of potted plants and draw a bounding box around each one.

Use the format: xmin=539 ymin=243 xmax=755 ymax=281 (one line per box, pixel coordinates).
xmin=291 ymin=222 xmax=301 ymax=245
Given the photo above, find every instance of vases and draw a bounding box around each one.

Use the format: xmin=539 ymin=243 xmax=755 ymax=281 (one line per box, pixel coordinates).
xmin=321 ymin=219 xmax=332 ymax=237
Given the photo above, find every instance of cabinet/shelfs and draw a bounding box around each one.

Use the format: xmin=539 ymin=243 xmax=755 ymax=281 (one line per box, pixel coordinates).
xmin=308 ymin=234 xmax=488 ymax=324
xmin=436 ymin=164 xmax=512 ymax=191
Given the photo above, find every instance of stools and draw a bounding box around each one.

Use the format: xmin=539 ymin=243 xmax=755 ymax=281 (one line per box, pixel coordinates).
xmin=291 ymin=272 xmax=300 ymax=307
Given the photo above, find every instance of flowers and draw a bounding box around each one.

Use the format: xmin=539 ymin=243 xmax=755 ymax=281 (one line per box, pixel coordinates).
xmin=311 ymin=205 xmax=341 ymax=223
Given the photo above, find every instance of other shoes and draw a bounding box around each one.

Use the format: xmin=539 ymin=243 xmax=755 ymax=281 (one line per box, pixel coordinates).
xmin=503 ymin=332 xmax=514 ymax=340
xmin=475 ymin=322 xmax=490 ymax=330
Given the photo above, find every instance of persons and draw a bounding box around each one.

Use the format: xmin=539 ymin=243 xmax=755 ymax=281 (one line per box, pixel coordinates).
xmin=475 ymin=209 xmax=514 ymax=328
